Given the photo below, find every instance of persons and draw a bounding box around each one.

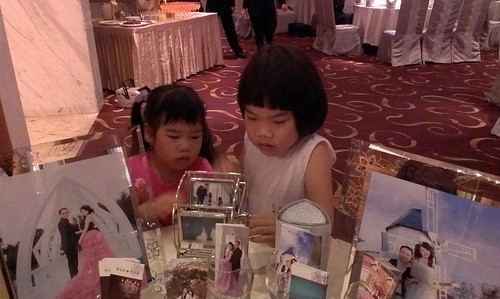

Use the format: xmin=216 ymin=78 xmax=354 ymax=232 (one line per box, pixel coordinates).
xmin=388 ymin=241 xmax=459 ymax=299
xmin=242 ymin=0 xmax=288 ymax=51
xmin=214 ymin=240 xmax=243 ymax=297
xmin=125 ymin=85 xmax=216 ymax=226
xmin=213 ymin=44 xmax=336 ymax=247
xmin=57 ymin=207 xmax=79 ymax=279
xmin=205 ymin=0 xmax=247 ymax=59
xmin=54 ymin=205 xmax=116 ymax=299
xmin=276 ymin=259 xmax=297 ymax=295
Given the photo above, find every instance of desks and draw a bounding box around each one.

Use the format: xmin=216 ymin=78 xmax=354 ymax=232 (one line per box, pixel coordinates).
xmin=92 ymin=10 xmax=227 ymax=90
xmin=98 ymin=219 xmax=353 ymax=299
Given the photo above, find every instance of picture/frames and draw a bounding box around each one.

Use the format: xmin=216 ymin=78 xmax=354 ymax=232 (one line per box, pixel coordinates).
xmin=188 ymin=176 xmax=235 ymax=210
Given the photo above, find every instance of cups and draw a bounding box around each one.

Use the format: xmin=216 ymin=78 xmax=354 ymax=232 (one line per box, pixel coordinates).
xmin=157 ymin=10 xmax=166 ymax=22
xmin=207 ymin=253 xmax=254 ymax=299
xmin=263 ymin=253 xmax=291 ymax=299
xmin=166 ymin=10 xmax=176 ymax=20
xmin=143 ymin=11 xmax=157 ymax=24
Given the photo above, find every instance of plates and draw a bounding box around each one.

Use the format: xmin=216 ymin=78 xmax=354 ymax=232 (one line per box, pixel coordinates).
xmin=119 ymin=21 xmax=148 ymax=27
xmin=99 ymin=20 xmax=124 ymax=25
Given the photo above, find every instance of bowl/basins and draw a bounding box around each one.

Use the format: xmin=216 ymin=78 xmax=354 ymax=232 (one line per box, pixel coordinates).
xmin=125 ymin=17 xmax=140 ymax=24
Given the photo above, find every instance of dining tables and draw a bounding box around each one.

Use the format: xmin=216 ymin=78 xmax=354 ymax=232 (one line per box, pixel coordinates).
xmin=352 ymin=3 xmax=434 ymax=46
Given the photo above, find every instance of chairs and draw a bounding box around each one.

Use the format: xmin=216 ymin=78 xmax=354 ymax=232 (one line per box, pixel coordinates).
xmin=378 ymin=0 xmax=500 ymax=66
xmin=312 ymin=0 xmax=361 ymax=58
xmin=231 ymin=0 xmax=296 ymax=37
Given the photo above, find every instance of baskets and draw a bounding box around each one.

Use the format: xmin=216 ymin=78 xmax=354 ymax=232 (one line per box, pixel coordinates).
xmin=115 ymin=78 xmax=142 ymax=109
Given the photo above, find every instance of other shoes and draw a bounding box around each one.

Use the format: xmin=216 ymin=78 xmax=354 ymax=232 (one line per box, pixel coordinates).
xmin=235 ymin=52 xmax=247 ymax=58
